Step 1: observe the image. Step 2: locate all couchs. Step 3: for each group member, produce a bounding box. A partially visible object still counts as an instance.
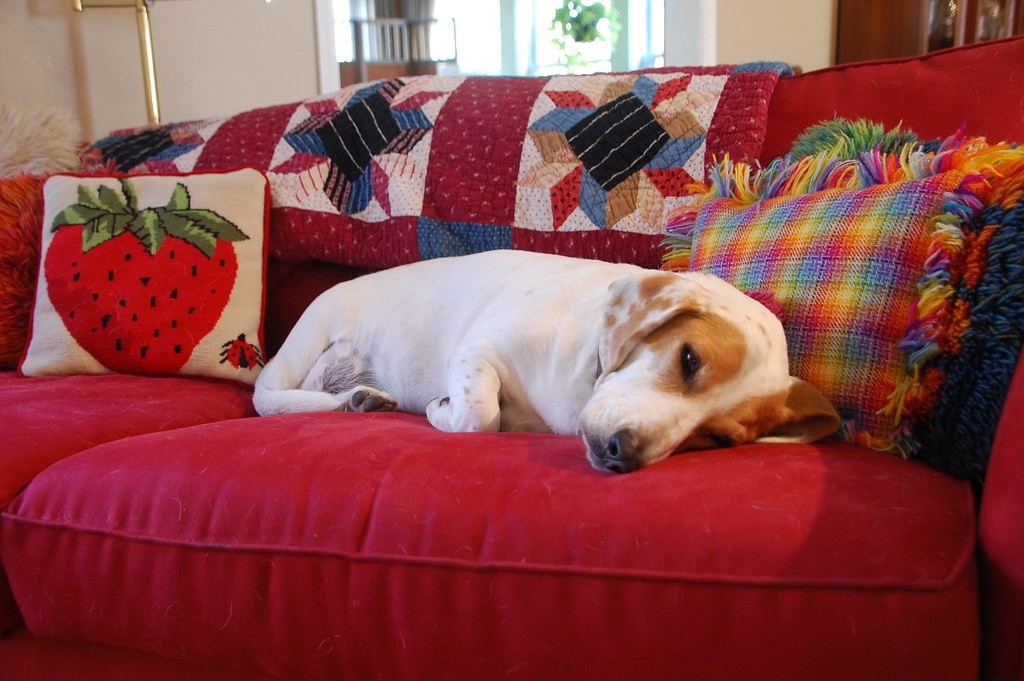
[0,36,1024,679]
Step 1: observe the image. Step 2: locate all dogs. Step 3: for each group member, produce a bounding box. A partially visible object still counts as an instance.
[251,249,841,472]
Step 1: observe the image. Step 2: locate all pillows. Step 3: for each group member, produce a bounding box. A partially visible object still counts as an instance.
[653,133,1024,457]
[18,170,272,394]
